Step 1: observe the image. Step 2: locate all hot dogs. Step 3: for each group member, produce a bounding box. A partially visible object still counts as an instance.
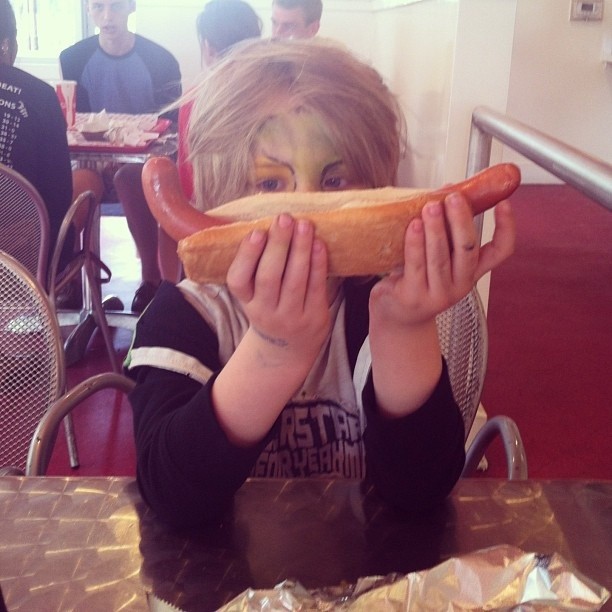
[142,158,519,285]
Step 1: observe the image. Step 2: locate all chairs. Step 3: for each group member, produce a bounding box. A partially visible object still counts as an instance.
[1,246,138,476]
[0,164,122,373]
[434,285,530,481]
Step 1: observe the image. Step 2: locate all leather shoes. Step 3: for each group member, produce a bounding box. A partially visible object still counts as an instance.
[131,278,162,313]
[56,280,84,310]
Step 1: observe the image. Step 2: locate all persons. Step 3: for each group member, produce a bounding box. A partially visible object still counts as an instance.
[270,0,323,40]
[57,0,183,312]
[196,0,264,69]
[0,1,75,301]
[124,38,516,509]
